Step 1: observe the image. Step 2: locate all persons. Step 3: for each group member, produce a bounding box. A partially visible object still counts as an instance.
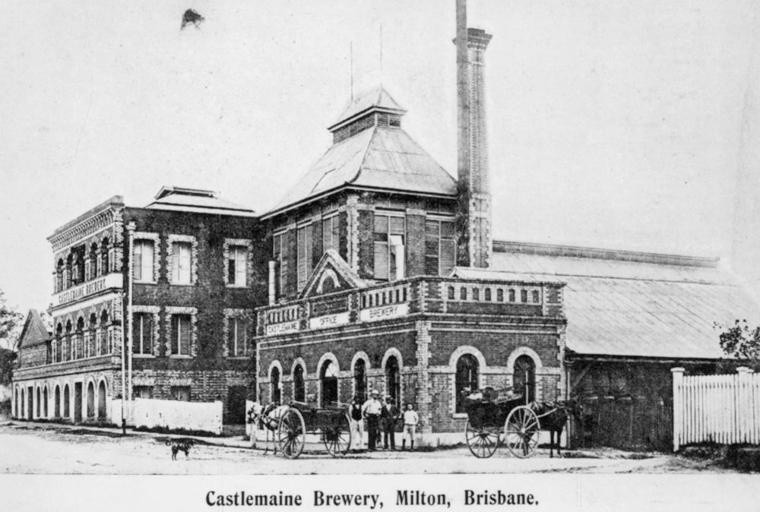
[362,390,383,452]
[402,403,418,451]
[349,396,364,451]
[381,395,400,451]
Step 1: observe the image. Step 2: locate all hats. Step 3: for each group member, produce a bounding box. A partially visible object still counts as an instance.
[386,395,393,400]
[372,390,379,394]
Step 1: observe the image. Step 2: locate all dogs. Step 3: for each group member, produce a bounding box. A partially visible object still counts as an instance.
[165,438,198,461]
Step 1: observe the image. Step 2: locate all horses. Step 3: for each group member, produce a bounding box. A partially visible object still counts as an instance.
[522,395,583,458]
[248,403,291,456]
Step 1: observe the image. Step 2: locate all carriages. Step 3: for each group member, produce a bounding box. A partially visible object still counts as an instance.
[245,397,353,458]
[460,382,582,459]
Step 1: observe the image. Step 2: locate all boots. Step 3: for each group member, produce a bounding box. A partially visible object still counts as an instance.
[411,440,414,450]
[402,439,405,450]
